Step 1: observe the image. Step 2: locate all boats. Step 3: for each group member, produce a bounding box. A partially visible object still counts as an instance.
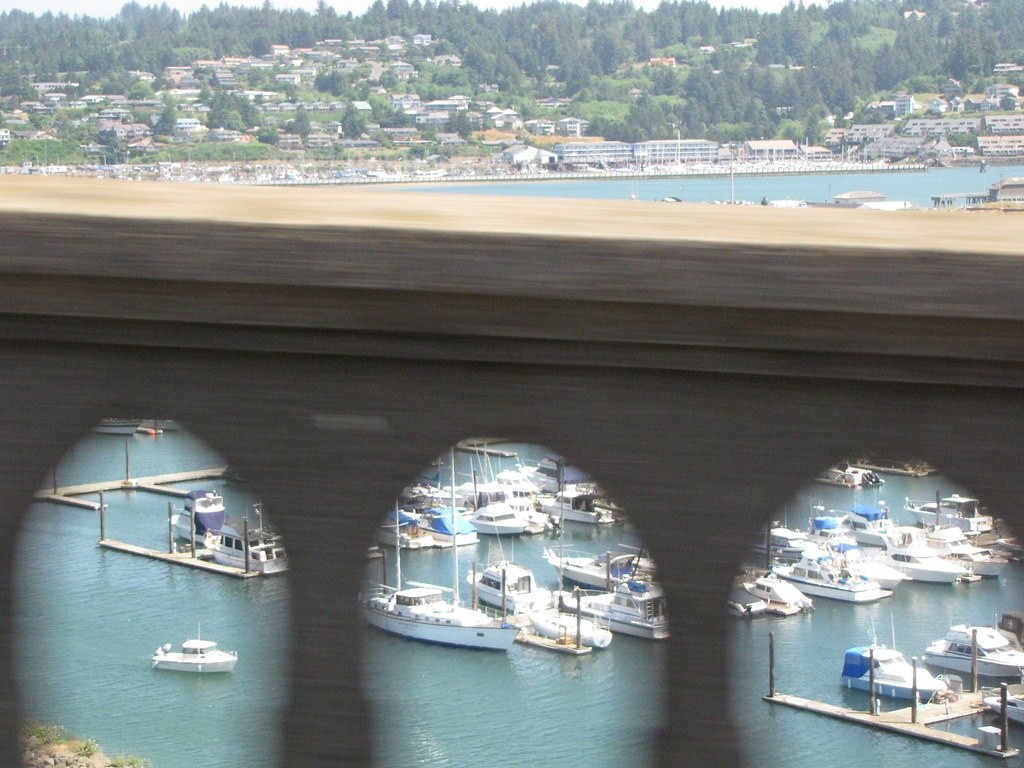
[730,453,1023,758]
[91,418,182,436]
[167,464,290,577]
[151,622,239,674]
[355,434,671,655]
